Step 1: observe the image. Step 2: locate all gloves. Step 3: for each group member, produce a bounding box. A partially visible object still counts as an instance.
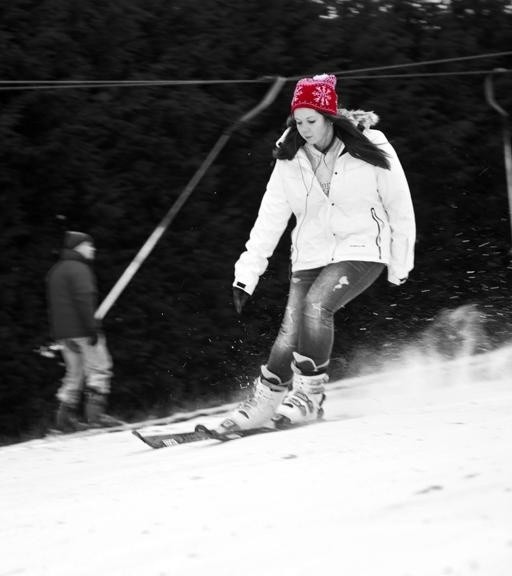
[231,288,249,314]
[85,330,97,346]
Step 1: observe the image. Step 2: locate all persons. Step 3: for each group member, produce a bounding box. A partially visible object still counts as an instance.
[39,227,126,438]
[219,68,420,436]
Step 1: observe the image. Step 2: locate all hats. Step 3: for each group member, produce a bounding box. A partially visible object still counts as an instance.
[291,73,337,116]
[62,230,91,250]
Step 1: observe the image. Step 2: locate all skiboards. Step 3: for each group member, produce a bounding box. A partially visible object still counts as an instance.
[132,417,328,449]
[42,404,228,447]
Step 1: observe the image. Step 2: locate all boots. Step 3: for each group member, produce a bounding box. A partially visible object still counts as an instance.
[214,364,292,438]
[80,392,125,425]
[271,351,331,429]
[45,400,86,433]
